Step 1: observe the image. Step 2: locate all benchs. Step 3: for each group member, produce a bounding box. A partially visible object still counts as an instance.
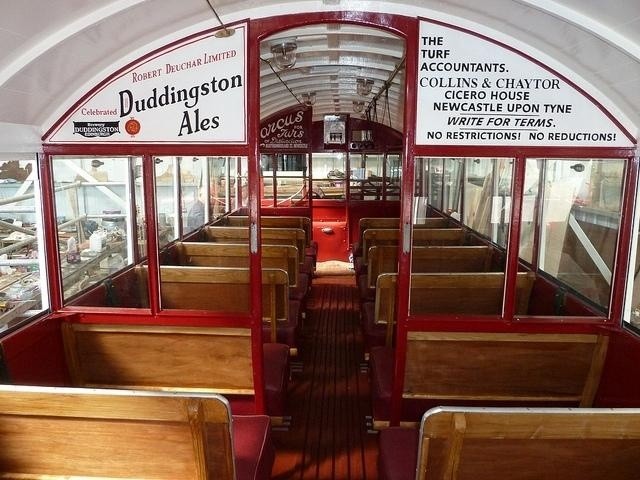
[368,316,619,424]
[374,403,640,478]
[0,379,277,476]
[349,217,494,300]
[129,260,303,350]
[200,215,319,281]
[61,301,295,420]
[358,270,536,343]
[173,238,309,306]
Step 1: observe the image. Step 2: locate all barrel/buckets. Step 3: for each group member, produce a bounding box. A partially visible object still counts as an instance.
[90,230,107,252]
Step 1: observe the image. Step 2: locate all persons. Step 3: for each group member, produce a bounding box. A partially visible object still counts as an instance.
[187,178,217,230]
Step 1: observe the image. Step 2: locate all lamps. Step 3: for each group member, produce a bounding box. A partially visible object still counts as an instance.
[355,77,374,97]
[269,40,297,69]
[300,94,315,108]
[354,102,364,114]
